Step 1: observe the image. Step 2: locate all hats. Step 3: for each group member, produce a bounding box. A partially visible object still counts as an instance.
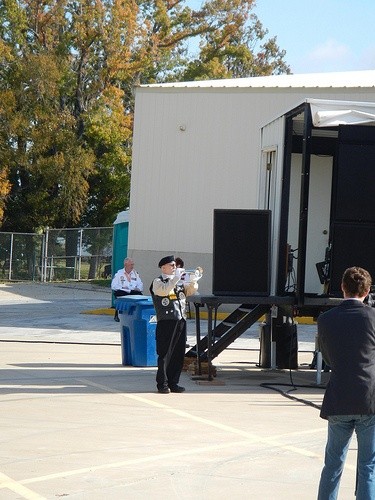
[158,256,174,269]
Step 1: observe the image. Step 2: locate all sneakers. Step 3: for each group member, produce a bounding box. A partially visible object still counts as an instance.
[158,386,171,394]
[173,386,186,393]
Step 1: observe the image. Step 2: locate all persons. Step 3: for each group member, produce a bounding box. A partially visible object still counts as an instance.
[174,257,190,348]
[317,266,375,500]
[111,257,143,322]
[149,255,203,393]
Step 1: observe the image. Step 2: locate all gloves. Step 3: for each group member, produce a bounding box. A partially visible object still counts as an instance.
[175,267,186,278]
[190,267,203,283]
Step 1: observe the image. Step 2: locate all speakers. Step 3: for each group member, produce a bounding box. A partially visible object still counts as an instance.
[259,323,298,369]
[327,124,375,298]
[212,209,272,296]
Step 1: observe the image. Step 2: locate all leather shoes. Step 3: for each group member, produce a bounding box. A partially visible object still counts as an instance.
[113,316,121,323]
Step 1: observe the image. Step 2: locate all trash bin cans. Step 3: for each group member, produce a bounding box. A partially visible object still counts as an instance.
[114,294,159,367]
